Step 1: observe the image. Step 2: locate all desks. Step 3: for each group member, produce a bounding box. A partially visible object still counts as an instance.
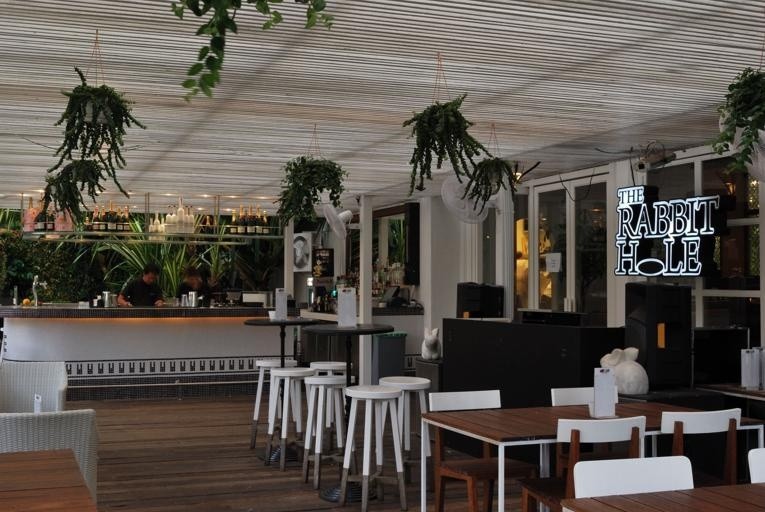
[242,318,321,463]
[561,483,764,512]
[302,323,394,503]
[2,449,97,511]
[422,402,763,501]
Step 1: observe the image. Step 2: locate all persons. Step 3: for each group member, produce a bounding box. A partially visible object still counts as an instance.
[117,263,165,308]
[175,265,206,306]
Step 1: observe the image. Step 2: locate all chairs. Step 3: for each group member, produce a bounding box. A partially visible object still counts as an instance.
[1,360,68,411]
[573,454,697,498]
[551,386,619,477]
[1,411,101,504]
[748,448,765,484]
[516,415,647,512]
[428,390,537,512]
[661,408,742,484]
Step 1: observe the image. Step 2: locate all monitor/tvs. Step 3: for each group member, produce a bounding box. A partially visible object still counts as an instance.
[379,286,400,303]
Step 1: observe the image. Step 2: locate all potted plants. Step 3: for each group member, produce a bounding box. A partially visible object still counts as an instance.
[402,51,522,216]
[37,31,151,232]
[273,124,351,226]
[229,206,270,234]
[703,37,765,182]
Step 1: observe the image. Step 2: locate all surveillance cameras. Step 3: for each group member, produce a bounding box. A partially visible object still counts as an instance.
[633,151,676,173]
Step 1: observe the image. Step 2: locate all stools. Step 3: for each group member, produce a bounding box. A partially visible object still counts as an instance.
[250,359,431,512]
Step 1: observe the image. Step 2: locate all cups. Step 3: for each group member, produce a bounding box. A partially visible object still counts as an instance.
[111,295,117,306]
[102,291,111,307]
[172,297,179,307]
[189,291,198,307]
[268,311,276,319]
[180,294,189,307]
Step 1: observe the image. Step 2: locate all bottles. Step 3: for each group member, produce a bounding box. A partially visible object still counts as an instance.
[149,205,194,233]
[84,205,130,231]
[312,294,333,313]
[229,203,270,235]
[33,209,55,230]
[334,272,383,296]
[205,215,212,241]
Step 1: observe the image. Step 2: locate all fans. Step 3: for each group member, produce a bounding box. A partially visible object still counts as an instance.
[440,176,502,224]
[321,203,353,240]
